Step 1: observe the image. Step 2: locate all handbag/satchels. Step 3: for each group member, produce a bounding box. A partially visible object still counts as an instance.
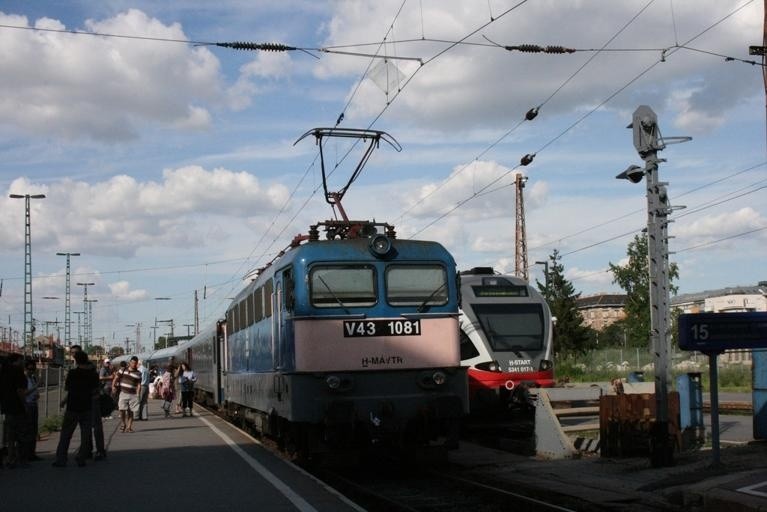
[110,386,120,401]
[100,394,116,416]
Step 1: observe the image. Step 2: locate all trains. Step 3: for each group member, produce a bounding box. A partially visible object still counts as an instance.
[108,219,468,467]
[455,265,555,412]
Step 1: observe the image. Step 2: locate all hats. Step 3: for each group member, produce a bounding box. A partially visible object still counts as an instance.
[104,359,110,363]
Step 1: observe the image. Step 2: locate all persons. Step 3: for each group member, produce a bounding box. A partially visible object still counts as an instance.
[608,378,625,395]
[1,346,198,467]
[228,334,275,373]
